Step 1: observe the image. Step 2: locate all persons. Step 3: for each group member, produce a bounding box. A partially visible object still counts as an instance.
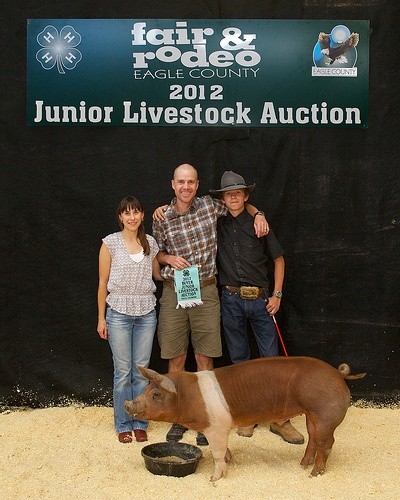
[97,196,164,443]
[153,171,305,444]
[151,164,269,445]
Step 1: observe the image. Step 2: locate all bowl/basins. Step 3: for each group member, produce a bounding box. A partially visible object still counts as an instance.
[141,442,202,477]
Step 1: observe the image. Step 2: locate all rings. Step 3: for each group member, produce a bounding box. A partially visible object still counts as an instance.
[264,228,267,230]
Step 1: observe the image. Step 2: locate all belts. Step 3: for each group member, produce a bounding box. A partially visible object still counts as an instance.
[223,285,269,300]
[164,278,215,287]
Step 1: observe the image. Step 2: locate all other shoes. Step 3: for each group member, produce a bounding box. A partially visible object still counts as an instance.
[270,419,304,443]
[118,429,133,443]
[238,424,256,438]
[134,429,147,442]
[167,422,188,442]
[197,430,208,446]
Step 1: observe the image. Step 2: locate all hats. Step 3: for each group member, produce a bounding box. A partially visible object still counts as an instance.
[209,171,257,192]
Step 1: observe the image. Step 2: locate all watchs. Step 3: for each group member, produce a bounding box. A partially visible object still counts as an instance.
[274,291,282,298]
[254,211,262,220]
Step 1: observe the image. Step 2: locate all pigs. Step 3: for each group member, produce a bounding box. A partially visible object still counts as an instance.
[122,356,366,482]
